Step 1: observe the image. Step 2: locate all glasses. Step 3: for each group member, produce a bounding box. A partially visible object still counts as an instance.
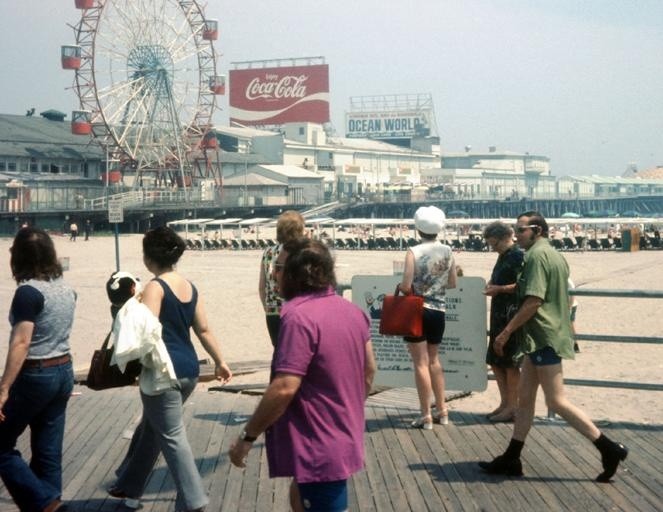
[518,225,537,232]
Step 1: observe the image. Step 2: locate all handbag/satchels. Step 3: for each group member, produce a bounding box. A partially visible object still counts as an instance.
[380,283,425,336]
[86,330,142,390]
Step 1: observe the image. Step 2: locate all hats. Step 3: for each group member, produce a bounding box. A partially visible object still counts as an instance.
[415,205,446,234]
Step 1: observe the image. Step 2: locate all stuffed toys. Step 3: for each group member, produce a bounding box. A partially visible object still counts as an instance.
[106,269,141,317]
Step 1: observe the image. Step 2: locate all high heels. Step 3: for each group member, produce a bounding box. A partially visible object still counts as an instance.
[412,409,448,429]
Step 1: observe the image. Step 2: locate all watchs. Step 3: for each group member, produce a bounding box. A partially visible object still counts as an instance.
[239,428,256,443]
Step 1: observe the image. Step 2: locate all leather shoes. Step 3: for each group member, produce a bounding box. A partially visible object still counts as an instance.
[597,442,627,481]
[479,456,522,474]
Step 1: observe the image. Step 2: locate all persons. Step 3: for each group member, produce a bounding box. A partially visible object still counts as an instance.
[481,212,631,483]
[550,218,661,245]
[214,231,218,243]
[204,229,208,241]
[229,243,374,512]
[481,222,528,425]
[67,220,78,240]
[0,226,77,511]
[256,209,310,350]
[405,202,458,432]
[108,228,232,511]
[83,220,91,240]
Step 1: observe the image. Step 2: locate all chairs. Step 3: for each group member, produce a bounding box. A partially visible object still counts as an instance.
[185,234,661,252]
